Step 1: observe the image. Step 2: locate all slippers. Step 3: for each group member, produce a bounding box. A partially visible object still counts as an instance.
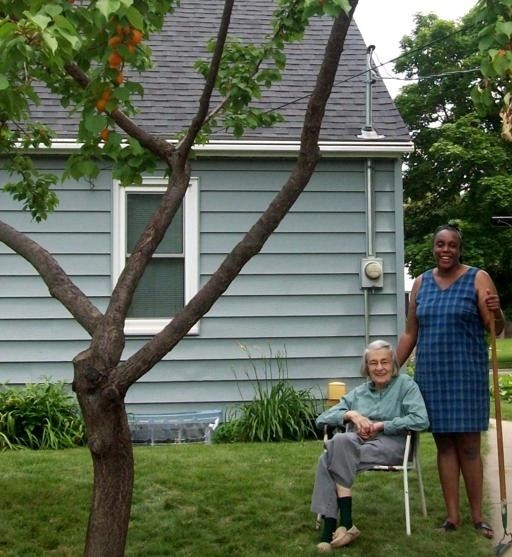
[472,521,493,540]
[439,520,462,534]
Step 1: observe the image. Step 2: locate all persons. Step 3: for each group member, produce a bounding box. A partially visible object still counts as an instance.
[395,224,505,539]
[310,340,430,551]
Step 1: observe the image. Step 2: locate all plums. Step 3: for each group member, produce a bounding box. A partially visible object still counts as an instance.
[95,23,142,141]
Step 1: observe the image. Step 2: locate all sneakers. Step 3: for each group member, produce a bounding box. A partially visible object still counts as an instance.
[317,524,362,553]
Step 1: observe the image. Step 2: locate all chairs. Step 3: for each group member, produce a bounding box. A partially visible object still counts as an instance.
[315,420,427,537]
